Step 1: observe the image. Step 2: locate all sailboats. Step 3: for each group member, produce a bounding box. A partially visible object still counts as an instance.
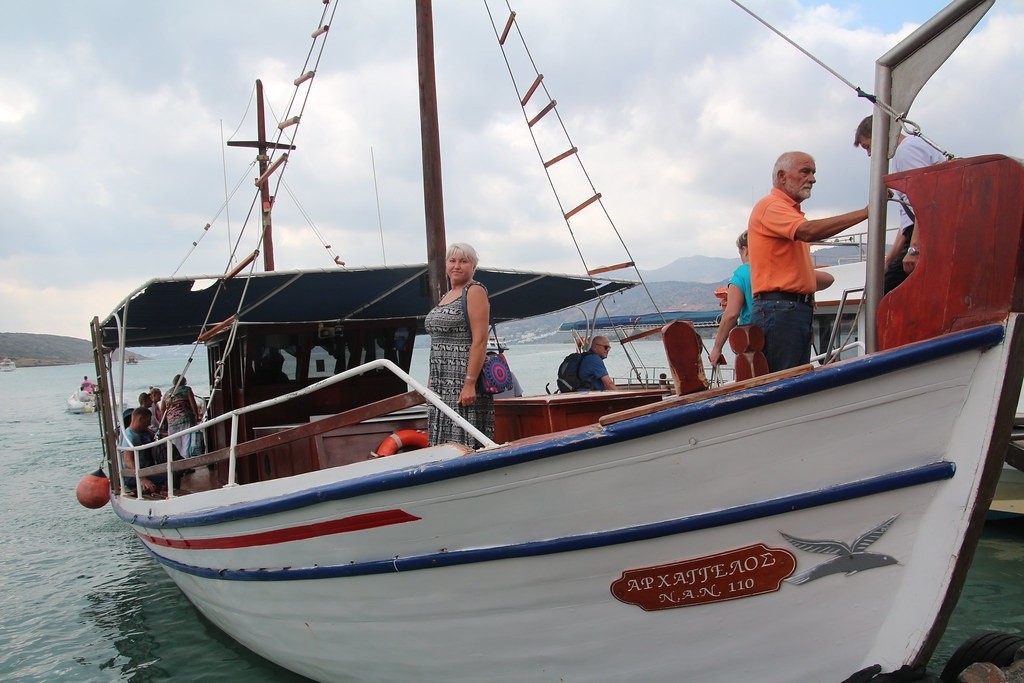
[73,0,1023,682]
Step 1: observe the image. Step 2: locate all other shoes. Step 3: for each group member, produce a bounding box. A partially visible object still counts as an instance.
[186,468,195,474]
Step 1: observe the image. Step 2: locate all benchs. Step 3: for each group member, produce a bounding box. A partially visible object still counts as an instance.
[136,484,189,500]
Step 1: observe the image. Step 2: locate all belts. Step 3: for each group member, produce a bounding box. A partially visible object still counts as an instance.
[754,290,814,307]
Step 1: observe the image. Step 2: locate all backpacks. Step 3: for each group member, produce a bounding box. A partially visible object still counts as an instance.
[557,352,596,393]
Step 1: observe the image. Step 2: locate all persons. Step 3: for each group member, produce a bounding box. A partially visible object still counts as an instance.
[557,336,616,393]
[81,376,92,393]
[855,115,946,294]
[709,230,834,367]
[747,152,894,373]
[79,387,90,402]
[120,375,199,494]
[486,352,524,398]
[425,243,494,452]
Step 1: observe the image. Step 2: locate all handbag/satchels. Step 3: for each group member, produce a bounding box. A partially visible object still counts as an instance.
[152,442,185,465]
[476,353,513,395]
[188,420,205,456]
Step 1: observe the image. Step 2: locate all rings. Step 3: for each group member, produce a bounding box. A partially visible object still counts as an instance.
[471,401,473,403]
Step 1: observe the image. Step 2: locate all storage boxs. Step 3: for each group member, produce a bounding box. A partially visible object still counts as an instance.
[252,414,433,481]
[493,390,670,444]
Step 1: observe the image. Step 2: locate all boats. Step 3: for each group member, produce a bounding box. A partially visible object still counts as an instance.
[66,391,98,415]
[0,357,17,373]
[557,220,1024,529]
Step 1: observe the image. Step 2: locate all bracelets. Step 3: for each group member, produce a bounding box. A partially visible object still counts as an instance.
[465,376,477,380]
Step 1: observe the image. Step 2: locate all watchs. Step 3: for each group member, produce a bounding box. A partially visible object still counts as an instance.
[908,248,920,255]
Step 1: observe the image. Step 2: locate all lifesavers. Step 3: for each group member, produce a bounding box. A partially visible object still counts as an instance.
[375,428,429,458]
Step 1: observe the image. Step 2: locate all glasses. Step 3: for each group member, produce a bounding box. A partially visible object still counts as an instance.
[596,343,611,350]
